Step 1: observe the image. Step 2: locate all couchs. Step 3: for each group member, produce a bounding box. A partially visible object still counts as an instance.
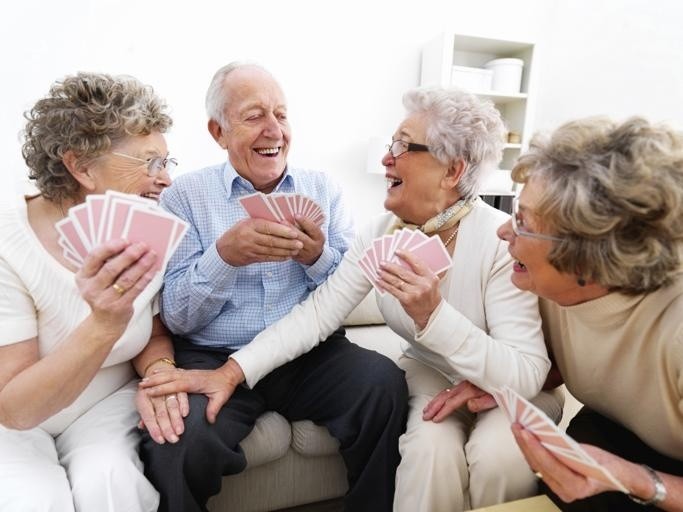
[209,287,401,512]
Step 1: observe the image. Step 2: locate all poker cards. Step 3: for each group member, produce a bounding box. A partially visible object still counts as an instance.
[358,227,453,296]
[54,189,190,276]
[236,193,327,227]
[489,386,629,493]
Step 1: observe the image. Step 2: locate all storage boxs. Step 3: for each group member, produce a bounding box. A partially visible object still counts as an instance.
[451,66,492,93]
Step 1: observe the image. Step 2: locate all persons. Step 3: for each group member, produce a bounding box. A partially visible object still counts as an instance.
[1,70,190,512]
[137,59,408,512]
[137,82,553,512]
[422,116,683,512]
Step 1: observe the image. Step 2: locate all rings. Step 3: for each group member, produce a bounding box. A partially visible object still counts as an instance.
[398,281,405,289]
[532,471,543,479]
[112,283,125,294]
[177,368,184,372]
[166,395,176,402]
[444,387,451,392]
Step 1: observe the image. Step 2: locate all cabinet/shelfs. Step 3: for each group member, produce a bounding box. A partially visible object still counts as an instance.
[421,31,536,212]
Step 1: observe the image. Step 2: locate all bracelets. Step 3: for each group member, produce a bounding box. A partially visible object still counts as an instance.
[144,357,176,373]
[627,464,666,506]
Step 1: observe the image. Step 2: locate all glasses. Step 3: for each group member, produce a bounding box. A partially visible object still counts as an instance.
[108,149,180,177]
[386,139,430,157]
[510,196,563,245]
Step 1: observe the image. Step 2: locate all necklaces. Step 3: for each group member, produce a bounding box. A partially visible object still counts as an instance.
[444,226,458,247]
